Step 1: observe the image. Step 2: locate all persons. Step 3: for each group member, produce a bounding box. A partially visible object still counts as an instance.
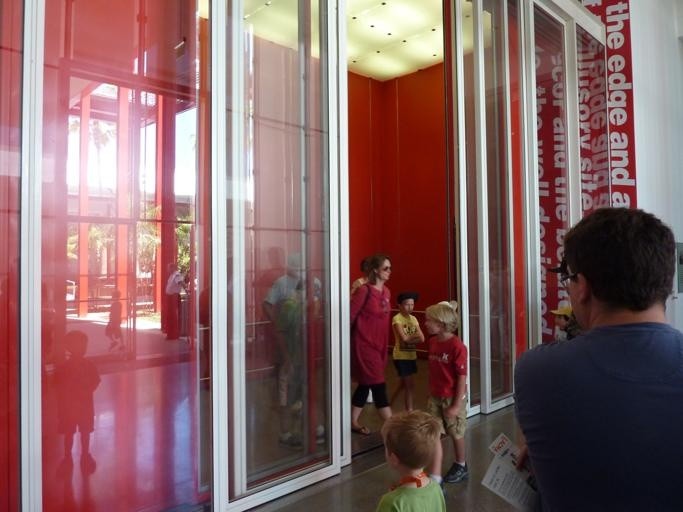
[515,207,682,512]
[548,256,580,345]
[52,330,101,472]
[160,263,191,342]
[350,254,468,512]
[101,291,131,351]
[261,242,322,445]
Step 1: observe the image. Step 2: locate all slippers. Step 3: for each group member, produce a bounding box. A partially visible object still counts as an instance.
[352,425,371,436]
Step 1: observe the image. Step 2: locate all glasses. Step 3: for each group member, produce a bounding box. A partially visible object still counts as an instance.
[381,266,392,272]
[559,273,577,294]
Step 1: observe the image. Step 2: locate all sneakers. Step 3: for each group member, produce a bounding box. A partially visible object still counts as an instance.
[366,390,372,404]
[443,461,469,483]
[108,341,125,351]
[55,453,96,473]
[439,482,447,499]
[278,428,326,449]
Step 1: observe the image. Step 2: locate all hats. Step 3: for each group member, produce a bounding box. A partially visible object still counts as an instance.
[397,292,419,304]
[549,305,572,319]
